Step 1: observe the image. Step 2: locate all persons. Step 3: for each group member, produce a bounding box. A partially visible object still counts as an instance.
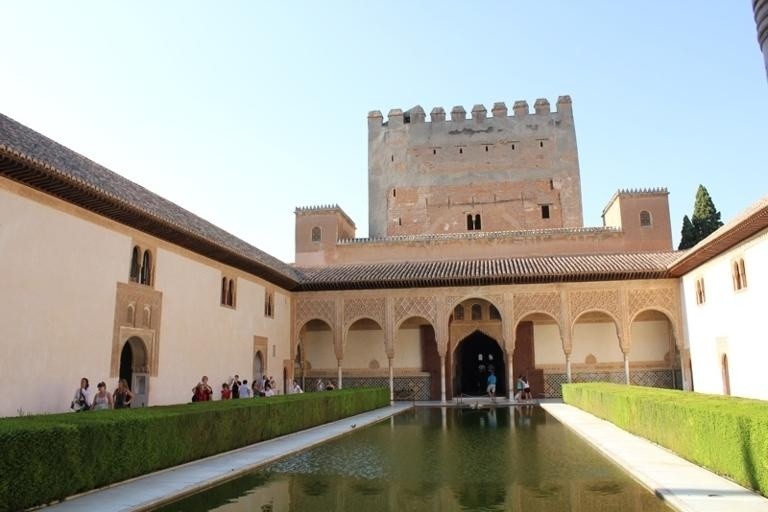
[317,379,324,392]
[291,380,303,394]
[522,376,533,400]
[70,377,93,411]
[191,373,276,403]
[113,379,135,408]
[326,380,334,391]
[90,382,114,410]
[514,375,526,400]
[486,372,497,399]
